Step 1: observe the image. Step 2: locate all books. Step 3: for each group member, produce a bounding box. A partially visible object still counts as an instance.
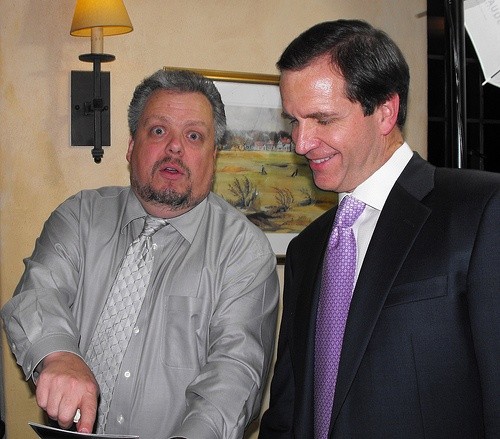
[28,421,140,439]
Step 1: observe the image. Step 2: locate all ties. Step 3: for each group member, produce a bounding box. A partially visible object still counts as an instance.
[314,195,365,439]
[79,217,169,436]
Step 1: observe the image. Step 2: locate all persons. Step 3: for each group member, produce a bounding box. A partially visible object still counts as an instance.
[259,20,500,439]
[0,70,280,439]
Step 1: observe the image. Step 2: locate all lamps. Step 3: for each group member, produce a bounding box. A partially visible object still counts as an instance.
[70,0,133,163]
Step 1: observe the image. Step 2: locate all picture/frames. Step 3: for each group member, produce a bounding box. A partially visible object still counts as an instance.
[164,66,339,265]
